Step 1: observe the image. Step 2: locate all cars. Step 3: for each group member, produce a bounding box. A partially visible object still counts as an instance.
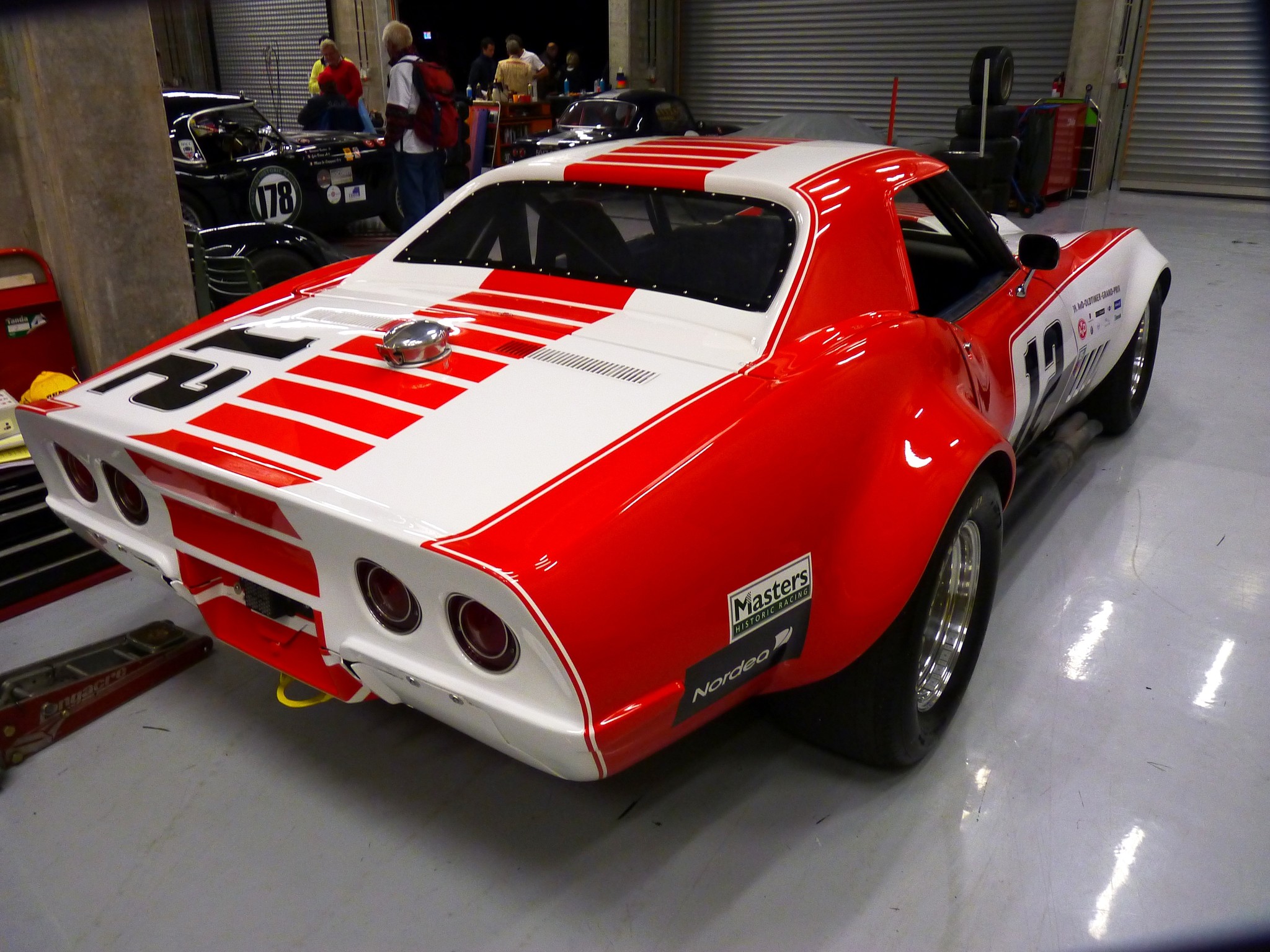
[513,89,742,162]
[186,219,354,319]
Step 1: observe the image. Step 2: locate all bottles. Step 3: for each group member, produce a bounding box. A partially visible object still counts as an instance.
[476,82,482,97]
[466,84,472,100]
[564,77,570,95]
[1052,72,1066,98]
[600,78,605,92]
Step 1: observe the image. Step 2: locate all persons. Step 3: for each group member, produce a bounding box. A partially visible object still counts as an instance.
[317,37,363,111]
[307,35,357,97]
[556,52,591,95]
[534,41,561,102]
[464,38,498,102]
[294,73,366,133]
[489,40,534,103]
[381,20,446,238]
[504,35,549,103]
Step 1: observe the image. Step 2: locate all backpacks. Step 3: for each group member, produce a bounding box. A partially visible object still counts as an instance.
[396,56,462,149]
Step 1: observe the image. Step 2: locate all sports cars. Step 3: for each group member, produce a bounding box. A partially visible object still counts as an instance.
[15,133,1175,790]
[163,89,443,242]
